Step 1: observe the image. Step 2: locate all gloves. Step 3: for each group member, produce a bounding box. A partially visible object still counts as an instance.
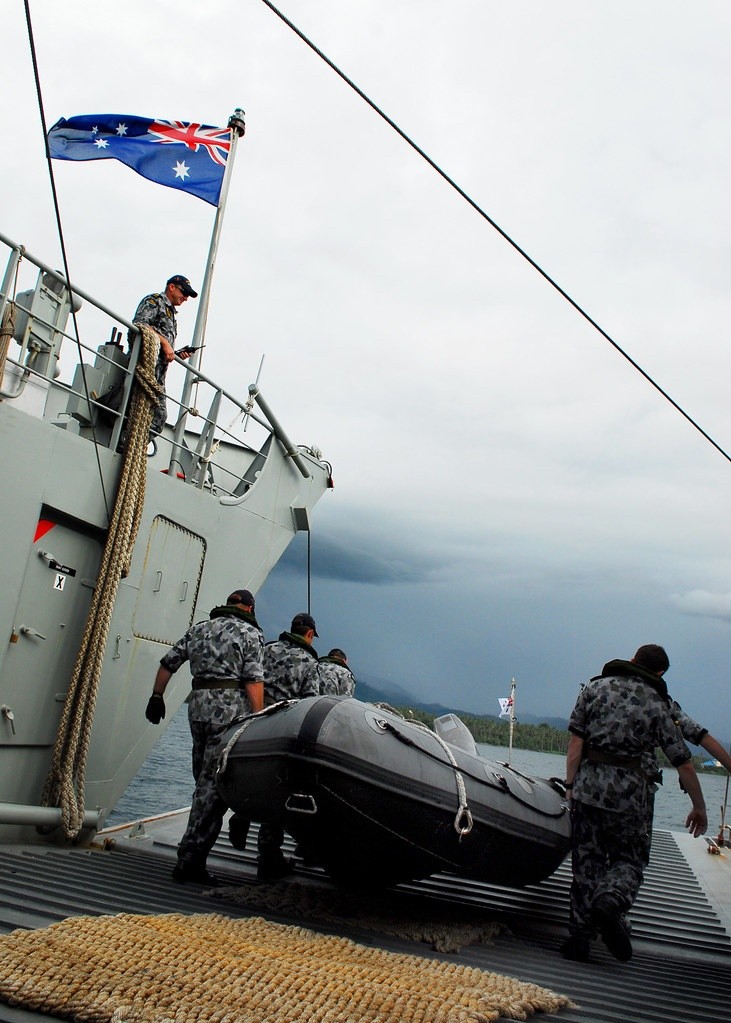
[146,691,165,725]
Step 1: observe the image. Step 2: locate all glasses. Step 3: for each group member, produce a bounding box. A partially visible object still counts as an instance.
[175,285,189,297]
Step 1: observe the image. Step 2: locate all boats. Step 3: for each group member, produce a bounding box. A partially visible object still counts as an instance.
[212,687,572,888]
[0,100,343,849]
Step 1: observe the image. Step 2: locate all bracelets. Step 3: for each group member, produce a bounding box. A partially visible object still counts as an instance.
[565,782,573,789]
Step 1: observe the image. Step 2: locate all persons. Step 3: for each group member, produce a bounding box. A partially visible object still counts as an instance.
[144,589,358,885]
[565,643,731,963]
[117,274,198,453]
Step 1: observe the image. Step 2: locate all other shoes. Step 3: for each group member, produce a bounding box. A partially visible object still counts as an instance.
[589,899,632,962]
[561,936,591,963]
[258,854,294,878]
[228,814,250,850]
[172,853,218,885]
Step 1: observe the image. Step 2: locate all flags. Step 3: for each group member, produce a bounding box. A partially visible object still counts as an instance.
[497,697,513,718]
[44,112,232,207]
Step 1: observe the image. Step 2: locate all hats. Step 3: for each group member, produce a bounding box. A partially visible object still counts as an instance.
[167,275,197,298]
[292,613,320,638]
[228,590,255,615]
[329,649,346,661]
